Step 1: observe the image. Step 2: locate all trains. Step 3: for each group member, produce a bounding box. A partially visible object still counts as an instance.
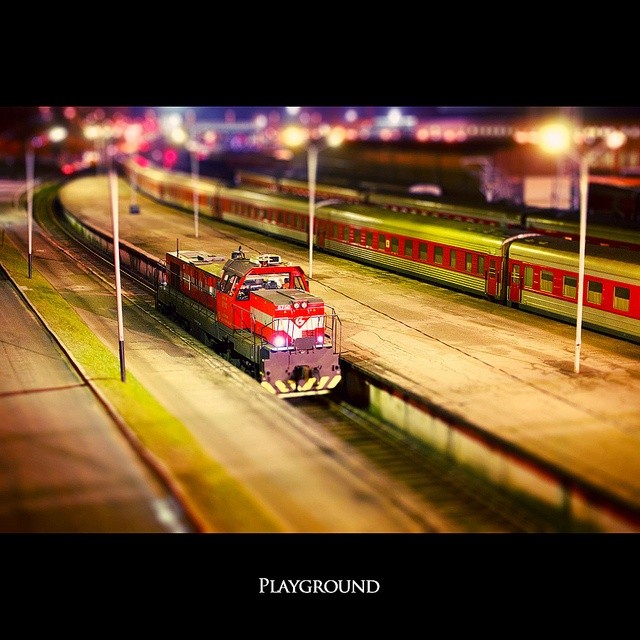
[157,240,343,401]
[114,151,639,345]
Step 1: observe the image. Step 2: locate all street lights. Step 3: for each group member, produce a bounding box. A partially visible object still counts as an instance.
[23,124,69,279]
[172,130,208,236]
[83,127,142,383]
[281,121,348,278]
[538,119,626,375]
